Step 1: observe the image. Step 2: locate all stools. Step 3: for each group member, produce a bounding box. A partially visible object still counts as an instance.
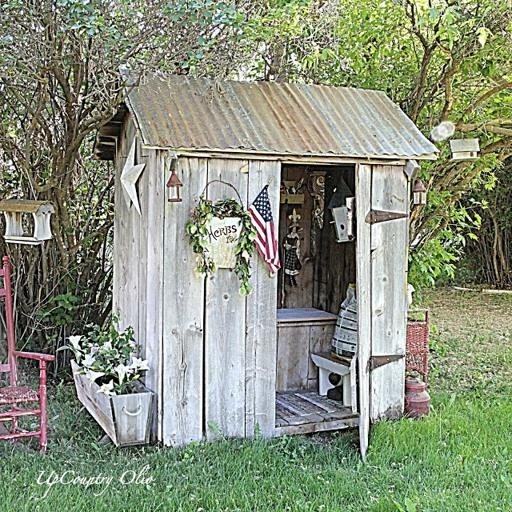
[308,351,351,410]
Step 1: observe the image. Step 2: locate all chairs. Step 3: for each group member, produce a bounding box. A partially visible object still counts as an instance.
[0,255,55,454]
[405,306,430,385]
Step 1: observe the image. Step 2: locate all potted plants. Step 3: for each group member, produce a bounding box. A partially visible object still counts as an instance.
[183,194,259,299]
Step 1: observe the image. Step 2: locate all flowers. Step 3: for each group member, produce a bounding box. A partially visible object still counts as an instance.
[54,307,150,397]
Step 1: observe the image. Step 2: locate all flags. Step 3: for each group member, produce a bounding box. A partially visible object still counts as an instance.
[244,186,282,276]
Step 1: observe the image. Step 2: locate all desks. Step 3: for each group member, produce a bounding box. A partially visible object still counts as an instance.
[277,306,338,393]
